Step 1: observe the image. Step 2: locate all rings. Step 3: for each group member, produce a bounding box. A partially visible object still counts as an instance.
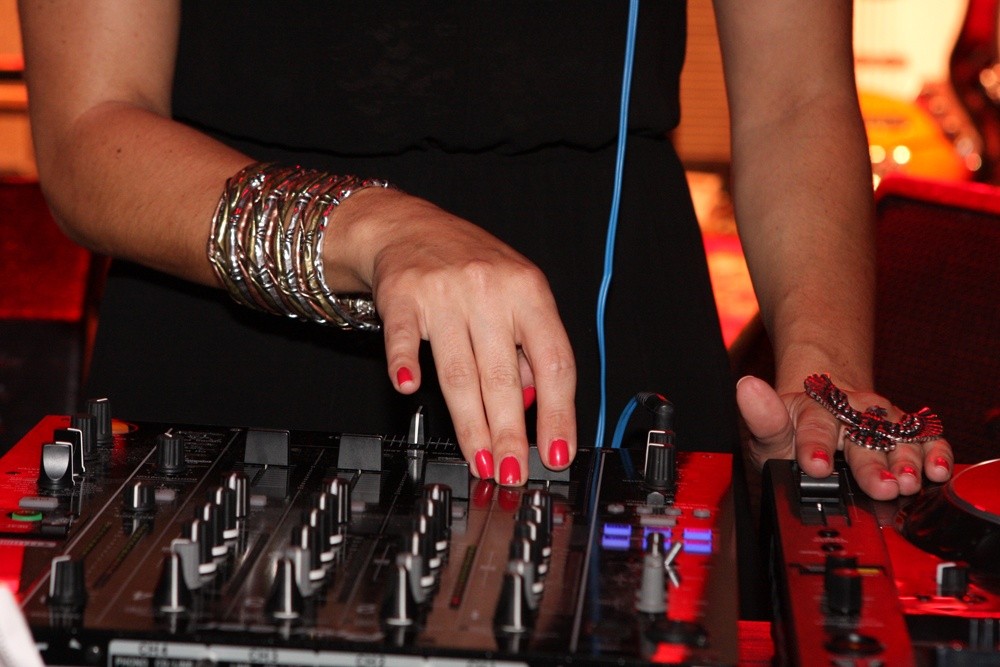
[803,372,943,452]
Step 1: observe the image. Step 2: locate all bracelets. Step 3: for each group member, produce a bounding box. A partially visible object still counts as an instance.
[206,161,404,332]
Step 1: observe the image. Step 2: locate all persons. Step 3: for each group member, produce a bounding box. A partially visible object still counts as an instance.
[15,0,954,500]
